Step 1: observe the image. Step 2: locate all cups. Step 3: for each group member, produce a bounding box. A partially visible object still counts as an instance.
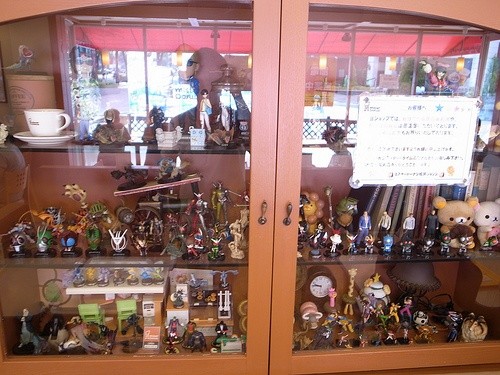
[24,108,71,137]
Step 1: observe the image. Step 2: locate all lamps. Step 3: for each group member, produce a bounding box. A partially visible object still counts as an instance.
[387,261,442,310]
[101,49,110,66]
[177,50,182,66]
[247,52,253,69]
[320,54,327,69]
[456,39,464,72]
[391,56,397,70]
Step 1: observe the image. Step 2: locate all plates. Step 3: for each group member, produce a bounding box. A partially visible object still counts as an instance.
[13,130,78,144]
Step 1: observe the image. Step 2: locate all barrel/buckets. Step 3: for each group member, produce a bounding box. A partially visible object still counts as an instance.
[5,74,57,132]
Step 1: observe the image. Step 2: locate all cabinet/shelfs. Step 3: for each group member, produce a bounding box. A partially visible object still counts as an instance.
[0,0,500,375]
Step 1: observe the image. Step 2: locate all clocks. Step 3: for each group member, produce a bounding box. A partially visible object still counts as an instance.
[300,265,336,310]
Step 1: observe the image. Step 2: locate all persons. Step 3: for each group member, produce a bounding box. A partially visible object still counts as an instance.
[199,89,213,132]
[321,127,352,168]
[188,192,208,235]
[308,210,437,251]
[312,297,412,347]
[169,317,181,333]
[211,182,235,224]
[165,47,249,222]
[215,321,228,339]
[228,208,249,253]
[423,64,447,89]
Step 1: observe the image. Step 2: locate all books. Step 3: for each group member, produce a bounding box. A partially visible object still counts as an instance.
[346,156,500,244]
